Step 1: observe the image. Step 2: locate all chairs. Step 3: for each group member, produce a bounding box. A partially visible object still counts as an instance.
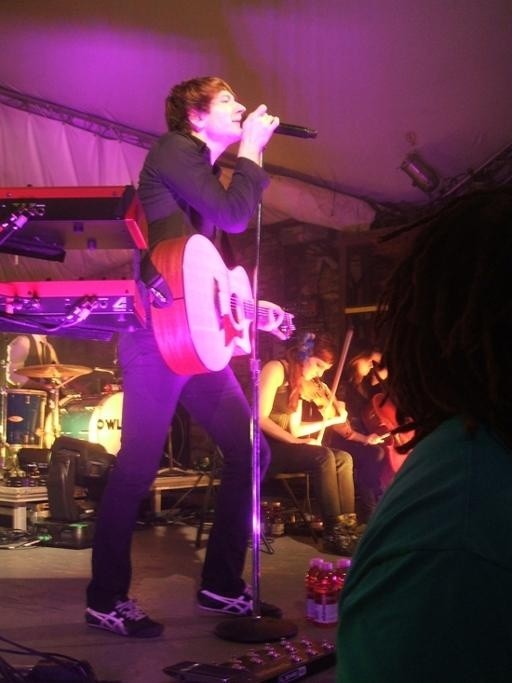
[195,434,321,554]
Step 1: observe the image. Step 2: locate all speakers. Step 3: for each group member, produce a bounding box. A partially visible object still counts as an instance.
[159,402,190,468]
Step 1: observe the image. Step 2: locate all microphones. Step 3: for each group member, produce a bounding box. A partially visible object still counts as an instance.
[238,115,319,139]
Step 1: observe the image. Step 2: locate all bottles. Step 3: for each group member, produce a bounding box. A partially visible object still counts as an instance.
[302,555,351,626]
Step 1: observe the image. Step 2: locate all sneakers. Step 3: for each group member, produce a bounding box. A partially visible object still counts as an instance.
[198,588,281,618]
[85,597,162,637]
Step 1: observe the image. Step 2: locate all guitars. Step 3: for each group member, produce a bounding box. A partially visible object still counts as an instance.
[146,235,293,377]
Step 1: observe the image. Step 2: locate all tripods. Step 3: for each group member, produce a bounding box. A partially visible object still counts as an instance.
[157,421,193,476]
[195,446,274,554]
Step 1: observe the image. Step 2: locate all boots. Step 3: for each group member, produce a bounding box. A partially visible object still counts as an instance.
[324,513,363,554]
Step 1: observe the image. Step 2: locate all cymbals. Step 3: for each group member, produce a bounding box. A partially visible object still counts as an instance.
[13,363,92,380]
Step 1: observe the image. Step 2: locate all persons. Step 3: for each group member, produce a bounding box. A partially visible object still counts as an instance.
[337,189,512,683]
[85,77,284,637]
[319,336,385,524]
[6,331,60,449]
[259,335,363,556]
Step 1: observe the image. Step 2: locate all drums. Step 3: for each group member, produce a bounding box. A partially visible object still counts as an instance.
[49,392,126,458]
[1,389,47,451]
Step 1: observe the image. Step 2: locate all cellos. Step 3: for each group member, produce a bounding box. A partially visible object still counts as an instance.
[357,342,424,492]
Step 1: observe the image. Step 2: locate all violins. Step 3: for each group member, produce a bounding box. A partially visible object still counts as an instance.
[302,379,347,422]
